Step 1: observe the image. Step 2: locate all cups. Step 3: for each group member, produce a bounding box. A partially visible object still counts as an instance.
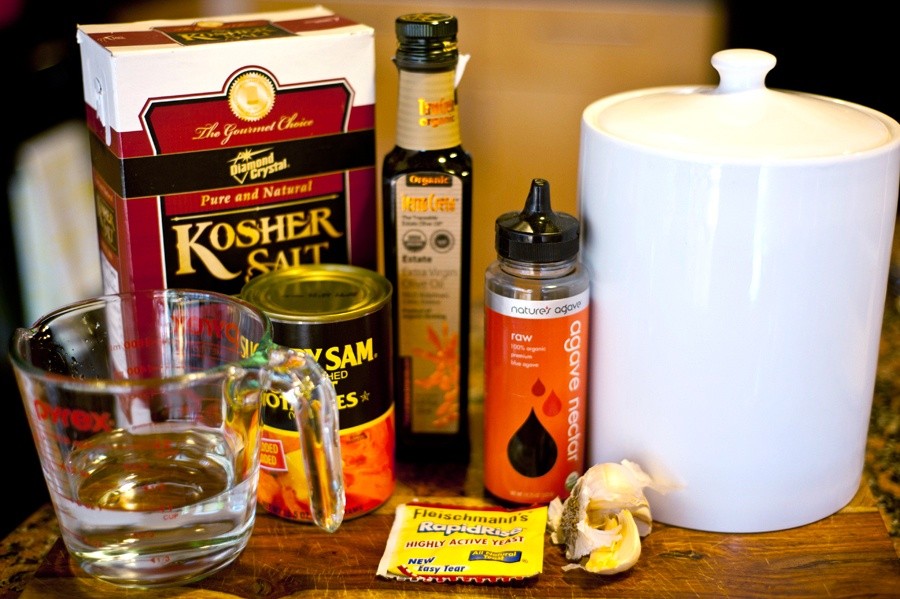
[8,288,345,592]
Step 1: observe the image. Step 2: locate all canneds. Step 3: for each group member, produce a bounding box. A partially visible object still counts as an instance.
[243,264,396,527]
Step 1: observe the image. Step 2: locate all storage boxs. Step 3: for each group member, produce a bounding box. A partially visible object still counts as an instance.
[77,5,379,426]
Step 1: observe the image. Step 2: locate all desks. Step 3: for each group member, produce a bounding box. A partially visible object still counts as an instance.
[1,408,900,599]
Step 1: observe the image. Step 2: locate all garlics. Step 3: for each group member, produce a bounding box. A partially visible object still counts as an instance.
[553,462,654,575]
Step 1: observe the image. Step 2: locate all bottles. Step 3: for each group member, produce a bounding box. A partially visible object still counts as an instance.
[577,48,900,535]
[484,177,591,503]
[380,10,473,461]
